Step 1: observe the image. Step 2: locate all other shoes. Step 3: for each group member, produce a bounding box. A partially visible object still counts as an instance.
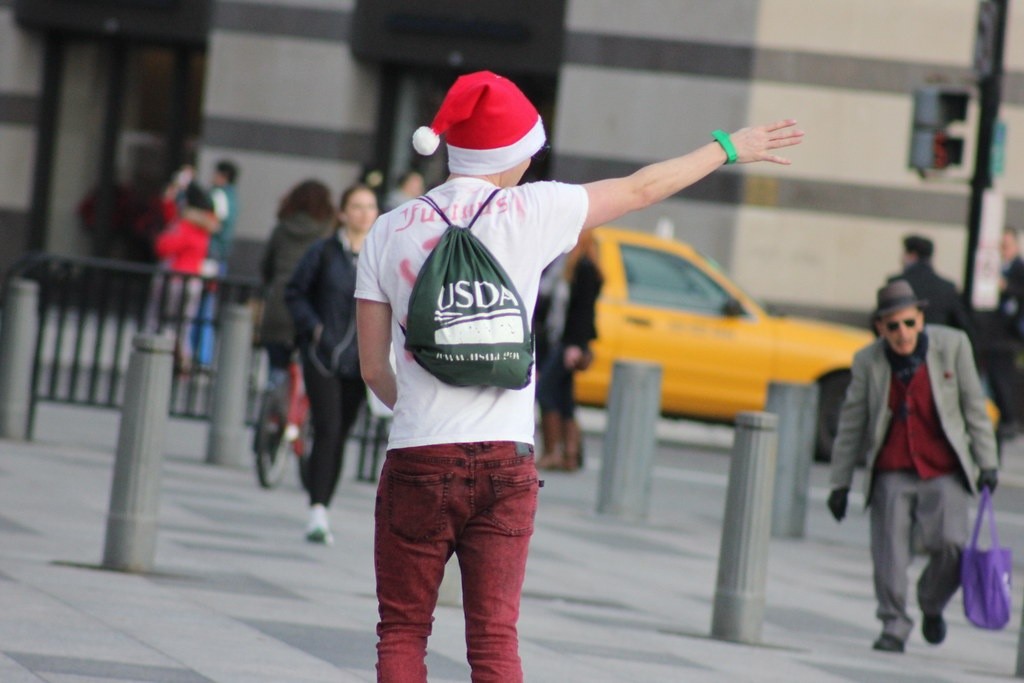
[923,607,947,643]
[305,502,335,545]
[873,634,902,652]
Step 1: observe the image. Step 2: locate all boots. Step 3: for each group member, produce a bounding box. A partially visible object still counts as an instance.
[563,418,580,471]
[534,412,564,472]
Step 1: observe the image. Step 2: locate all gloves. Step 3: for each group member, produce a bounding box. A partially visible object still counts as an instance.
[977,465,999,497]
[828,488,847,525]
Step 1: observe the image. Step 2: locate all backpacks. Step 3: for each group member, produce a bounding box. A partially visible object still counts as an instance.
[401,192,533,390]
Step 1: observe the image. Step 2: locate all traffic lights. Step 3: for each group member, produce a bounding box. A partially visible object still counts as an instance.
[911,82,970,174]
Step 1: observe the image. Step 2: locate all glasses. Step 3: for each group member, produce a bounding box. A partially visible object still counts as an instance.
[883,315,921,330]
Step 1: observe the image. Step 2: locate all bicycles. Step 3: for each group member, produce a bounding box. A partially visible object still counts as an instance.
[254,349,312,490]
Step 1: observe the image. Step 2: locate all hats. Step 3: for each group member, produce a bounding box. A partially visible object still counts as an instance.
[412,68,548,177]
[874,280,929,321]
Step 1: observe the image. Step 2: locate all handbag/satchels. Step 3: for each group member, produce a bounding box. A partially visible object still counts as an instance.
[958,490,1011,628]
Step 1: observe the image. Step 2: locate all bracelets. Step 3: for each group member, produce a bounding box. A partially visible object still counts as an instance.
[712,129,737,165]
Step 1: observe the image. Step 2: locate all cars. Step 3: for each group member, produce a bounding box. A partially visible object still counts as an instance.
[570,225,1002,469]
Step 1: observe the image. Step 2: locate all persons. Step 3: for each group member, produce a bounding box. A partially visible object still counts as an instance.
[998,226,1024,435]
[161,158,239,370]
[828,281,998,651]
[385,171,423,210]
[251,179,379,542]
[534,230,604,470]
[890,233,972,335]
[352,70,804,683]
